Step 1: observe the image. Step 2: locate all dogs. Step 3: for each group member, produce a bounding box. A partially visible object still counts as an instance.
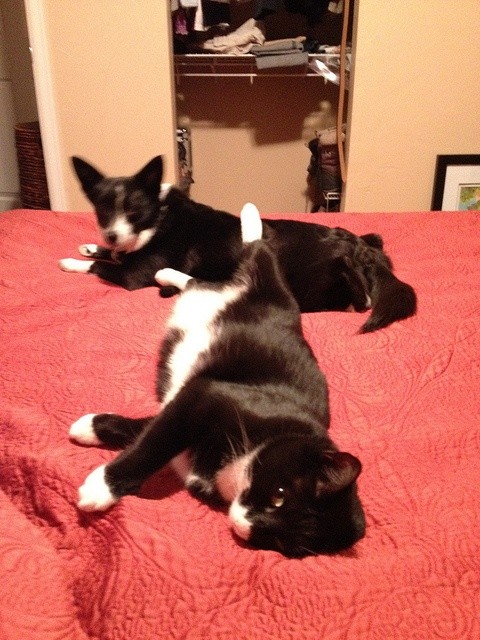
[57,155,419,338]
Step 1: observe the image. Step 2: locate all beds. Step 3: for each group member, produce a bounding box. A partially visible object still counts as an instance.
[0,207,479,640]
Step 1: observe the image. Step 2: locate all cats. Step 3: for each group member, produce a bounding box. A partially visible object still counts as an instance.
[65,202,367,559]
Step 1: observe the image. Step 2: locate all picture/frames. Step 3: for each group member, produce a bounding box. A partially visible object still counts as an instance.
[430,155,480,212]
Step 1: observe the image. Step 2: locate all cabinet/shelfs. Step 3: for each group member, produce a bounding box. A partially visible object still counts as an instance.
[175,48,353,82]
